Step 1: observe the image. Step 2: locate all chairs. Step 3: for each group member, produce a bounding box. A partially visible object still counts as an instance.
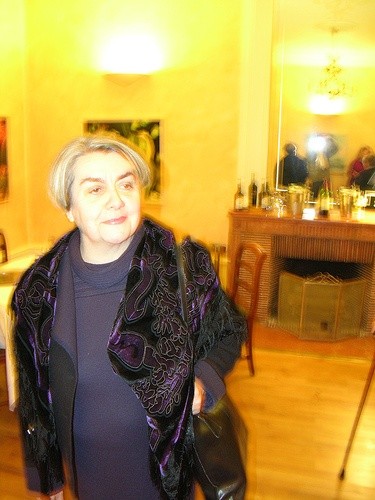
[182,235,266,375]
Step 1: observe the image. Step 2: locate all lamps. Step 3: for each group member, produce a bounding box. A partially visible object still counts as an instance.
[301,19,349,116]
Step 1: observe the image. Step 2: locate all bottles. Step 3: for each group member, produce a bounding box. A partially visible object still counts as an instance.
[320,179,329,216]
[234,179,244,212]
[248,173,258,208]
[259,183,270,209]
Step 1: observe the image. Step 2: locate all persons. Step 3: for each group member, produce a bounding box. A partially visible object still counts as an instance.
[314,150,333,198]
[10,138,251,500]
[355,154,375,190]
[275,143,308,188]
[346,146,370,188]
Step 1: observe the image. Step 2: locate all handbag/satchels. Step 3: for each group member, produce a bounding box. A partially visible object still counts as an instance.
[187,391,248,500]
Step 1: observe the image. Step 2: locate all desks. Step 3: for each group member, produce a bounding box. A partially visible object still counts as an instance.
[0,249,50,411]
[228,204,375,339]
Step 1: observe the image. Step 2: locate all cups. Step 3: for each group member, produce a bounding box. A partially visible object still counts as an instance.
[337,189,353,220]
[288,191,303,220]
